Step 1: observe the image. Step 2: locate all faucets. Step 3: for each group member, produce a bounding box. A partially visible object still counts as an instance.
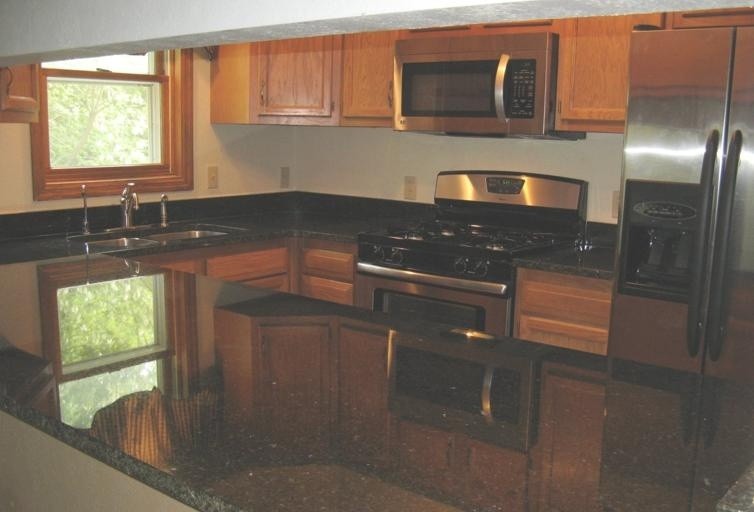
[125,193,140,215]
[79,184,89,221]
[160,194,169,225]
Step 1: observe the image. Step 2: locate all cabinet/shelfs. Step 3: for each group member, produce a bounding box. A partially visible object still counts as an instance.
[297,229,361,306]
[331,314,396,479]
[134,244,204,275]
[550,9,667,137]
[341,26,401,130]
[389,412,527,512]
[0,341,62,427]
[203,234,295,298]
[206,33,340,131]
[215,309,335,464]
[536,360,610,512]
[1,59,44,125]
[511,260,613,356]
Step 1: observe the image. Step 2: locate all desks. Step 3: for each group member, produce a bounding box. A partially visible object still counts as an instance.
[0,251,754,512]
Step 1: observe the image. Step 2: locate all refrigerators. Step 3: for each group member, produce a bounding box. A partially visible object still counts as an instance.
[596,358,753,512]
[611,28,753,381]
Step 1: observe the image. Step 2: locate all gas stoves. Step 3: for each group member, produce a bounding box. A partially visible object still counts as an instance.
[354,216,587,279]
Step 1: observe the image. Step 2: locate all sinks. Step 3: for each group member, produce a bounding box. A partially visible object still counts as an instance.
[147,229,227,239]
[86,237,159,253]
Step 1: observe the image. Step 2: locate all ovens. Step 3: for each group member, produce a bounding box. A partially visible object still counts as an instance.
[351,260,513,336]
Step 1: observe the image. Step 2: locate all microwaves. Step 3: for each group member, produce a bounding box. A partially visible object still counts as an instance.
[380,334,541,454]
[395,33,583,142]
